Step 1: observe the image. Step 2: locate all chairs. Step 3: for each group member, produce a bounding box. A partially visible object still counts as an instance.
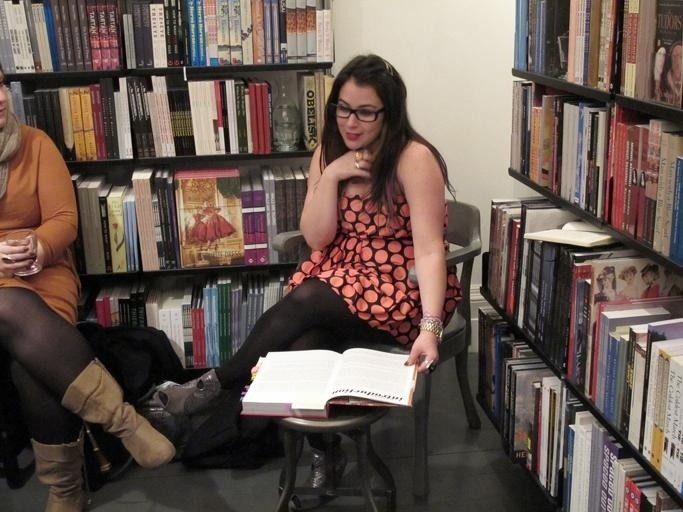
[0,175,96,489]
[272,202,482,501]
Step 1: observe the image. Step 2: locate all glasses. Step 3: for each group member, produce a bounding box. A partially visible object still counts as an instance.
[328,102,384,123]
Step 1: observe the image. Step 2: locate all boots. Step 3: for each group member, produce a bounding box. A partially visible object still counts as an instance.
[60,357,176,469]
[30,427,85,512]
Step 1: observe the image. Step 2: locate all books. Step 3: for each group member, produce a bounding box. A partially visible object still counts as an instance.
[491,195,680,489]
[240,348,421,417]
[1,0,334,70]
[478,307,683,512]
[74,271,294,366]
[10,73,335,159]
[496,76,683,261]
[69,159,310,267]
[513,0,682,107]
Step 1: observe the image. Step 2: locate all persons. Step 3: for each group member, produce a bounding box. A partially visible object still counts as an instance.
[1,67,182,512]
[154,54,464,512]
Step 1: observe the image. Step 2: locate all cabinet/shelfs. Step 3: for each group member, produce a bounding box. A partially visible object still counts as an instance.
[1,1,337,378]
[475,1,678,509]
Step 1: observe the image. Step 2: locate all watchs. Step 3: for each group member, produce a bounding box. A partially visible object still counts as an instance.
[420,319,444,344]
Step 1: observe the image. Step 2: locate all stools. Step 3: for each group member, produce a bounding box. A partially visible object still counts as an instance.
[275,405,400,509]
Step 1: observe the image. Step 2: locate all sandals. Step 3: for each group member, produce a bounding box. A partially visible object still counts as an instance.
[292,447,348,508]
[155,368,221,418]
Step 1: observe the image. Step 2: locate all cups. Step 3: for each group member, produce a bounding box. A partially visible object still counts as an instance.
[6,228,42,277]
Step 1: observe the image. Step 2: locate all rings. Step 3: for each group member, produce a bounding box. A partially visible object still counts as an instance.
[355,151,364,161]
[1,256,15,265]
[354,161,360,169]
[423,360,436,372]
